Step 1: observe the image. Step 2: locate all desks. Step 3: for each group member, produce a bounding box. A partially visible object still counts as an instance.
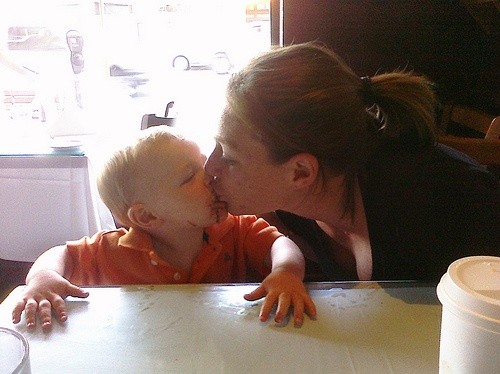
[0,279,443,374]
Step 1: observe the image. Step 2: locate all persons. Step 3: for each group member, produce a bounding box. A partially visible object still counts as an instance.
[203,44,500,281]
[11,125,317,328]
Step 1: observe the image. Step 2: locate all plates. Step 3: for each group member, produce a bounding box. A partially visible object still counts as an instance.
[49,142,83,151]
[0,326,30,373]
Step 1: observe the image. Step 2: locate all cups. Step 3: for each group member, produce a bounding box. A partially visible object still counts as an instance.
[436,256,500,374]
